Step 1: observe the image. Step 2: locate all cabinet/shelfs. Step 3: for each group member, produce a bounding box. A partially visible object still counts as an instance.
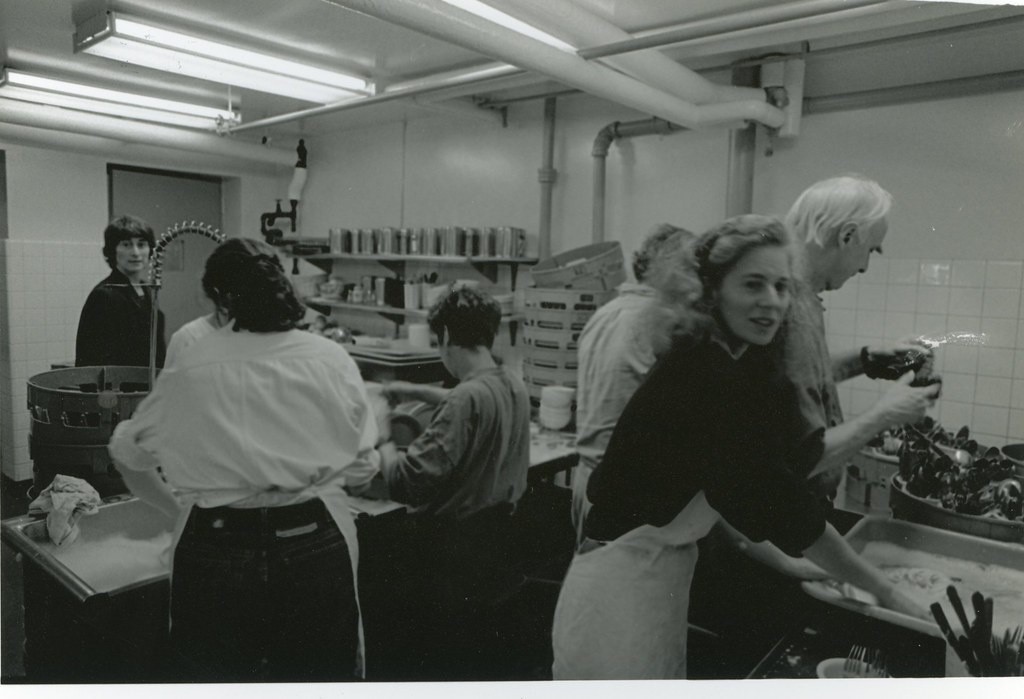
[302,254,540,350]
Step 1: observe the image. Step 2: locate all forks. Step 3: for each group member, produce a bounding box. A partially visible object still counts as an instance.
[842,645,866,677]
[931,585,1024,677]
[865,649,889,678]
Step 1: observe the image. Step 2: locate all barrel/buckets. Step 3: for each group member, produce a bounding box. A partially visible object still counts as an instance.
[841,450,901,514]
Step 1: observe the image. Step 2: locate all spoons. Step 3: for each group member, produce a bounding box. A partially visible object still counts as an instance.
[850,416,1024,522]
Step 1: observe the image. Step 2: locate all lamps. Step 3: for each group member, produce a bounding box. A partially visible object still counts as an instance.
[72,10,377,105]
[0,67,241,131]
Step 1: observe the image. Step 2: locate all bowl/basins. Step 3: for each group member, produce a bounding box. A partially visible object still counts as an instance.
[538,386,576,430]
[890,473,1024,543]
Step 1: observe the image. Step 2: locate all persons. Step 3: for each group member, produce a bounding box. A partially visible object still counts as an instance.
[696,175,940,679]
[366,282,532,682]
[107,238,382,683]
[74,214,167,367]
[568,222,697,547]
[549,216,935,679]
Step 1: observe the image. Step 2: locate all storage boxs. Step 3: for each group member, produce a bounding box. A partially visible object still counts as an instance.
[532,239,628,290]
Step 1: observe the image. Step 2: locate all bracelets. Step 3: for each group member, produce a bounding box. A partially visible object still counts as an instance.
[861,346,878,380]
[374,436,394,449]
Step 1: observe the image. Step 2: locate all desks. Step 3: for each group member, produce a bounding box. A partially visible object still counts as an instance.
[2,420,579,684]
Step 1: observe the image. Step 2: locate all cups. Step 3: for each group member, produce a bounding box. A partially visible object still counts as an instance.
[328,227,528,256]
[408,325,430,346]
[362,276,435,309]
